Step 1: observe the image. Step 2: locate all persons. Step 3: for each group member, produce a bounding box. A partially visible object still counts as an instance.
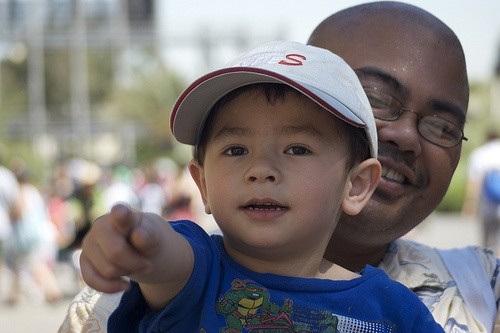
[0,134,225,310]
[80,41,434,333]
[55,0,500,333]
[458,124,500,256]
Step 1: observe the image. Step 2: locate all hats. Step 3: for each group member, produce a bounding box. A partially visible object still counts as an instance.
[169,39,378,160]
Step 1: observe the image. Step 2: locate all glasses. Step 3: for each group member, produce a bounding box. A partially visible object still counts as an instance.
[362,85,468,148]
